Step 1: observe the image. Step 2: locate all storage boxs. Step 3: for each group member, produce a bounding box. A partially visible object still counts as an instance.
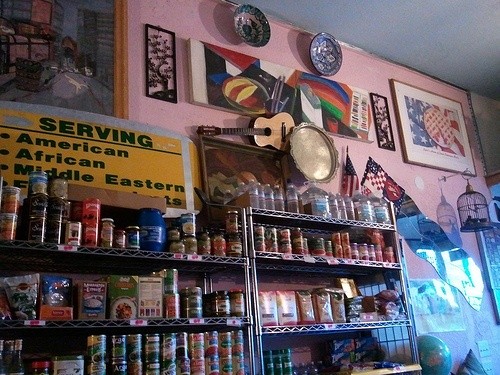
[38,274,163,319]
[259,290,296,326]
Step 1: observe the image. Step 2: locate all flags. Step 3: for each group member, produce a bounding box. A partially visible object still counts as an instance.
[343,153,359,196]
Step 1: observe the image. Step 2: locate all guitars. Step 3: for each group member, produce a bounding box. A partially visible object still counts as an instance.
[196,112,295,152]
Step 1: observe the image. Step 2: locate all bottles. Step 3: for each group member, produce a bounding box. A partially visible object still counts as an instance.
[138,207,168,252]
[221,178,392,224]
[262,348,324,375]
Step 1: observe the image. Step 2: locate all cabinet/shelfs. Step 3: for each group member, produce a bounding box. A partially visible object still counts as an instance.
[0,203,255,375]
[209,207,422,375]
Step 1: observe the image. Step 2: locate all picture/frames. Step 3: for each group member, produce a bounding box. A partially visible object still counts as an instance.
[198,135,290,210]
[390,78,478,177]
[0,0,130,120]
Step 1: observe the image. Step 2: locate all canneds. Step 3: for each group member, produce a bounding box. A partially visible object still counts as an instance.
[32,361,54,375]
[262,348,293,375]
[100,217,141,249]
[152,269,246,318]
[24,170,101,247]
[167,210,243,259]
[86,330,245,375]
[254,226,396,263]
[0,185,21,242]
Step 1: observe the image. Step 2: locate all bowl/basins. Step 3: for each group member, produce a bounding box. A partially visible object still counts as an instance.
[309,31,343,76]
[232,4,271,47]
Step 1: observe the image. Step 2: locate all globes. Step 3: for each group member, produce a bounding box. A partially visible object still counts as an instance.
[416,334,452,375]
